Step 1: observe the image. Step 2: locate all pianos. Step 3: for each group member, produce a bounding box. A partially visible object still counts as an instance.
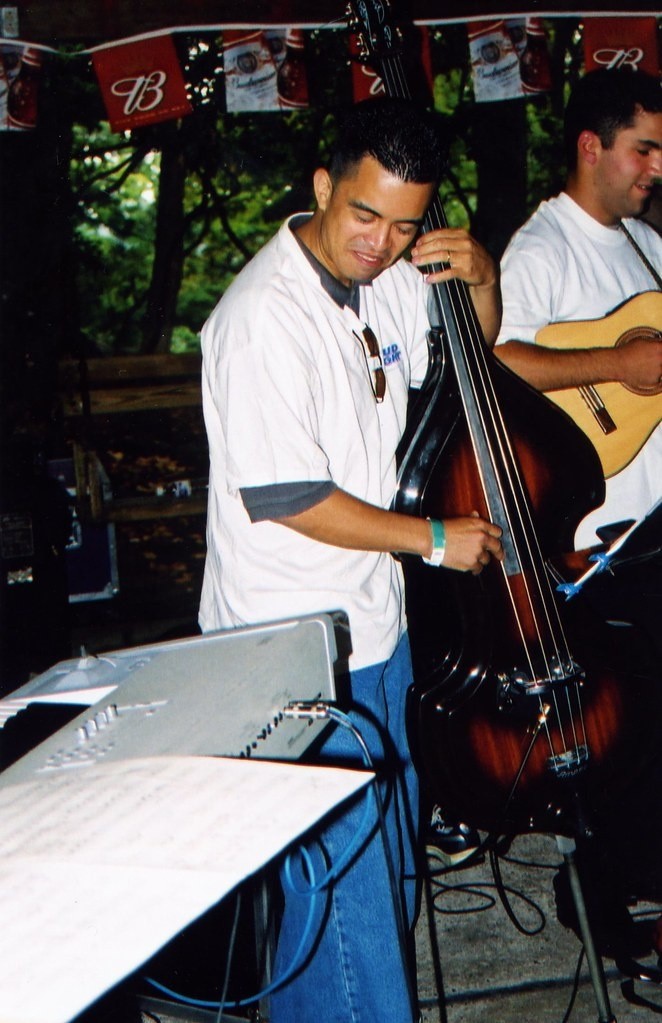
[1,611,348,1023]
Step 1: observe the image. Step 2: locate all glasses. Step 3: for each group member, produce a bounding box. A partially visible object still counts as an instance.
[352,323,386,404]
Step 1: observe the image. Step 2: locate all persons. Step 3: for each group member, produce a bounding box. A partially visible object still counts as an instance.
[491,66,662,957]
[196,96,504,1023]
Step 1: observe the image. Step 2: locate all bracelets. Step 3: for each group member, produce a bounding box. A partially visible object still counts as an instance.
[422,516,445,567]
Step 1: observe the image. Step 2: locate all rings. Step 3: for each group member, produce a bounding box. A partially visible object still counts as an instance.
[446,250,450,262]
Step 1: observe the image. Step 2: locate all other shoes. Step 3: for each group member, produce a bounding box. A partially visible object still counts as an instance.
[424,815,481,866]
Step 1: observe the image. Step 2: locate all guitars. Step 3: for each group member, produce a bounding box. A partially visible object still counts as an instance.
[526,286,661,478]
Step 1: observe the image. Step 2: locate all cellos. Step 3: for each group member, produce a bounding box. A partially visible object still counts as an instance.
[357,2,662,834]
